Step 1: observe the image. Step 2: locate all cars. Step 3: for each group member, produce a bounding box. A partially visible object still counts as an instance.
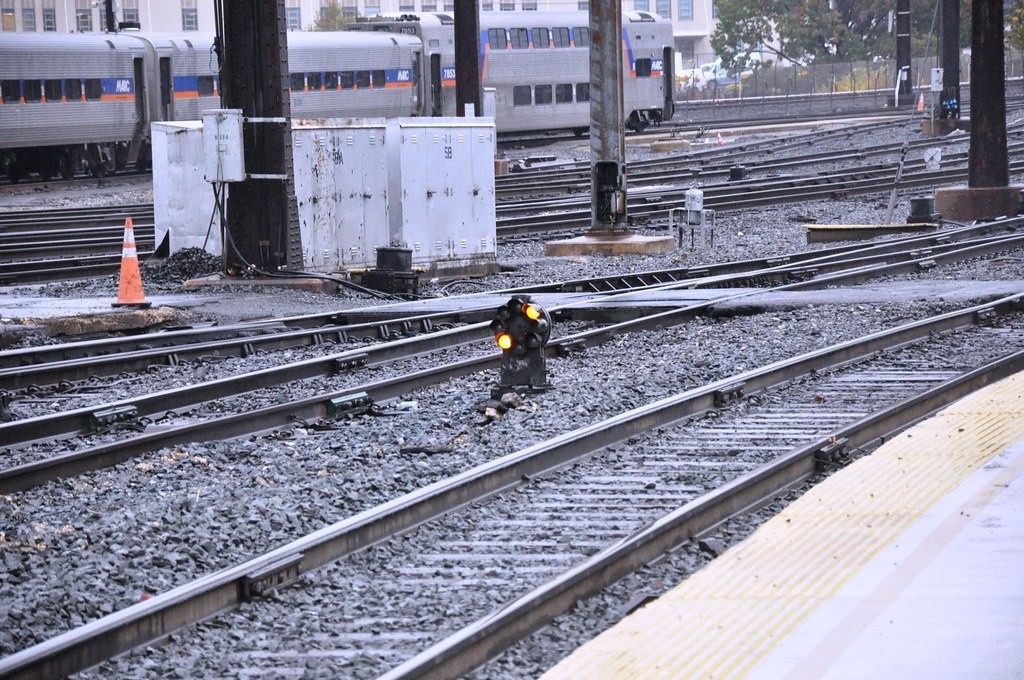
[679,63,711,89]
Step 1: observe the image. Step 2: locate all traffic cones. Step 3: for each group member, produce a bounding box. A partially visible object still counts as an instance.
[110,216,151,309]
[918,94,928,110]
[718,133,725,146]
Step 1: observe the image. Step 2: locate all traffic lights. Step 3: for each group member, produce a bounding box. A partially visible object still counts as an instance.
[491,297,551,356]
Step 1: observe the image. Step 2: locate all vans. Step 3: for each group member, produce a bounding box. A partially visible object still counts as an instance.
[704,52,762,93]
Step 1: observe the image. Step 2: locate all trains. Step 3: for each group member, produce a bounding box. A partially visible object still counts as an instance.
[0,9,677,182]
